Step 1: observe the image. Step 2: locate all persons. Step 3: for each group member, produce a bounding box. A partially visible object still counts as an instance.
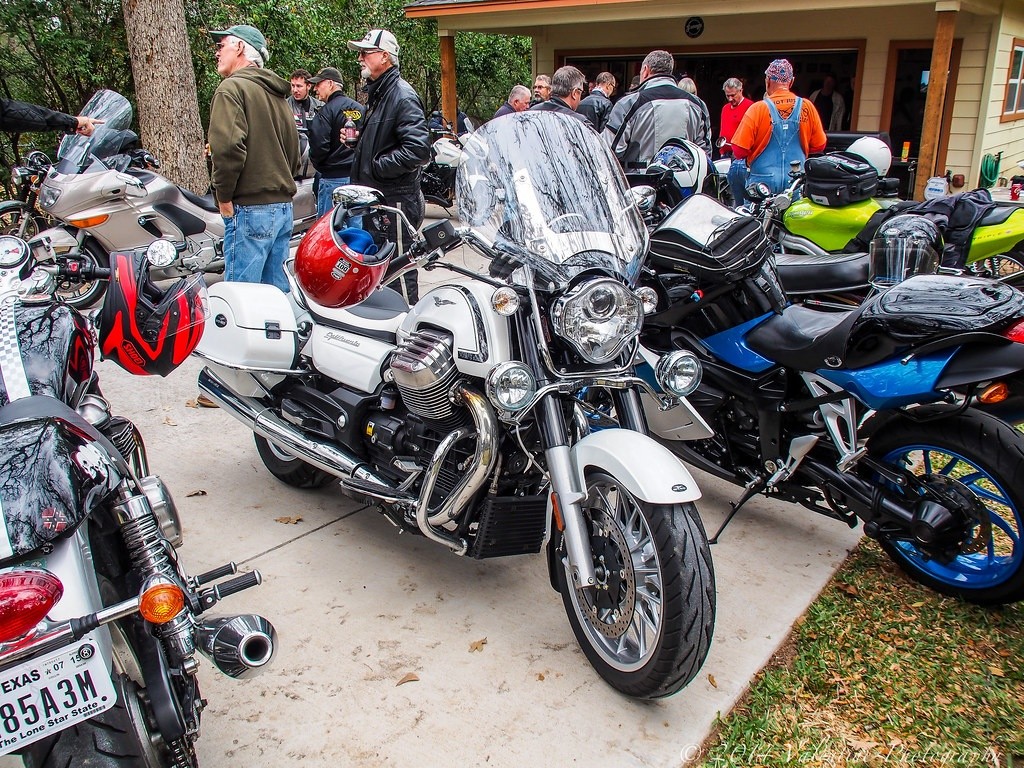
[718,77,754,161]
[731,58,828,208]
[282,68,325,181]
[206,25,302,293]
[307,67,364,232]
[494,71,856,222]
[0,98,106,138]
[340,28,432,307]
[600,50,712,199]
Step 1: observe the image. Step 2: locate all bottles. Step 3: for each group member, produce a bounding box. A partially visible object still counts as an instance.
[344,117,358,148]
[998,176,1007,187]
[901,142,910,162]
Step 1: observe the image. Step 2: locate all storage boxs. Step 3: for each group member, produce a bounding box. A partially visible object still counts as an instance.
[191,281,299,399]
[297,138,317,177]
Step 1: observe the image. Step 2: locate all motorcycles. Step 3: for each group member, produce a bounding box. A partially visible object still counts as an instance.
[13,89,355,310]
[3,187,280,768]
[610,130,1024,606]
[188,110,719,707]
